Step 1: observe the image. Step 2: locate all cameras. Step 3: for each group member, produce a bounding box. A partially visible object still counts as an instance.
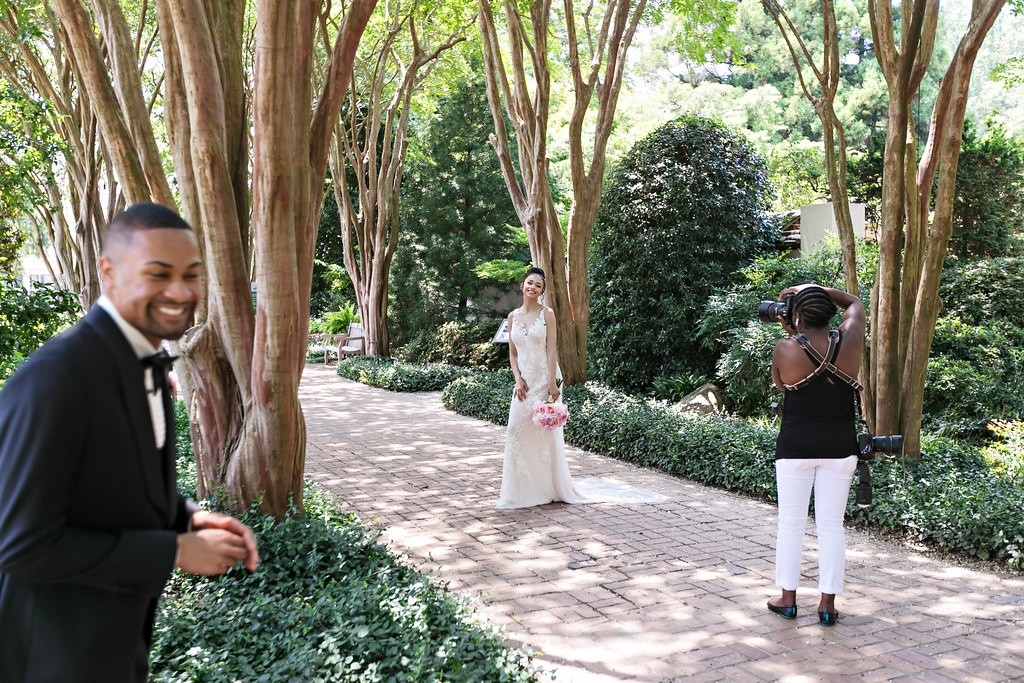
[857,432,903,508]
[758,294,797,327]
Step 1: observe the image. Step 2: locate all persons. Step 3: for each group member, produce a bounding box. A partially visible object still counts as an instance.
[496,267,576,509]
[767,283,866,626]
[0,202,258,683]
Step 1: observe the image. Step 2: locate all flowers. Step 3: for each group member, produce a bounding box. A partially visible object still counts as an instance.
[532,379,570,430]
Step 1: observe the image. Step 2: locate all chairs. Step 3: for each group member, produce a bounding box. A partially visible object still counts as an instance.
[320,320,365,365]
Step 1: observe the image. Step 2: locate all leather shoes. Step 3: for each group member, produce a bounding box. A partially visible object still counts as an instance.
[817,608,838,627]
[767,597,797,619]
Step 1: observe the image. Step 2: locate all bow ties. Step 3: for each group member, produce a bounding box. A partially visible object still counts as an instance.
[139,348,179,395]
